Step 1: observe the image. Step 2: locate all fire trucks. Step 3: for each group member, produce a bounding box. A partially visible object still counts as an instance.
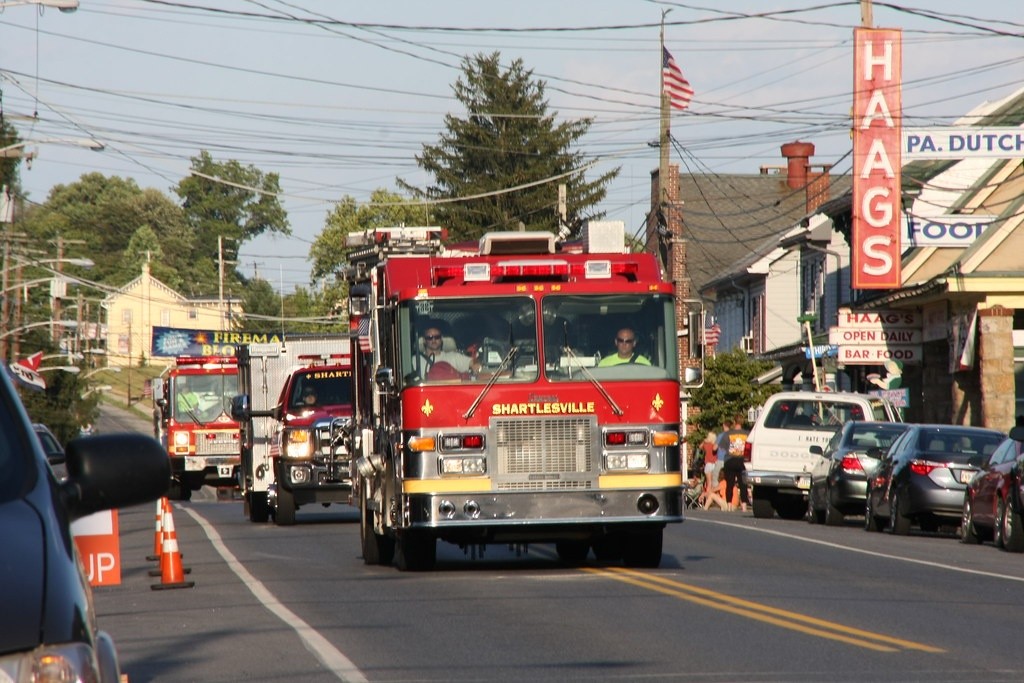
[348,224,700,573]
[230,338,358,527]
[149,355,239,501]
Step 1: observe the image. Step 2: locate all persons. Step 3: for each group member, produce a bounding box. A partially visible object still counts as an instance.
[413,325,480,382]
[177,382,200,419]
[295,386,320,408]
[597,329,652,370]
[698,414,750,512]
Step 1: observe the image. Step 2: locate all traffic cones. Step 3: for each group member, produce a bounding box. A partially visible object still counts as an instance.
[145,496,190,577]
[150,504,194,592]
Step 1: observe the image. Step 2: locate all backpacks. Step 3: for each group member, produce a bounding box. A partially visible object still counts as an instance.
[728,434,747,457]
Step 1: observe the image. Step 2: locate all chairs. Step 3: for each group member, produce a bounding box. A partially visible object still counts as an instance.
[418,337,456,354]
[854,438,876,447]
[928,440,945,452]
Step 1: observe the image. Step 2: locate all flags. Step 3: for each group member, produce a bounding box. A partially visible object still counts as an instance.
[701,311,721,347]
[358,319,375,352]
[663,44,695,111]
[8,352,47,396]
[143,379,152,395]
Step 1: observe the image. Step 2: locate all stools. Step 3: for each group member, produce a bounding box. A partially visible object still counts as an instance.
[683,488,702,510]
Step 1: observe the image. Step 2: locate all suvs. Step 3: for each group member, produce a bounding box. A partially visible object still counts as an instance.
[742,390,903,521]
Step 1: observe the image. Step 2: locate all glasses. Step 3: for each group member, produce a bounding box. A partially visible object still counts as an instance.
[425,334,442,341]
[616,337,634,343]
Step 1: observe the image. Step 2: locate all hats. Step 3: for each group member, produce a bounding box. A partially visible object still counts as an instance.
[304,388,317,396]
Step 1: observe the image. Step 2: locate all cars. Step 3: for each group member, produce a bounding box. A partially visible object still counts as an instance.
[30,421,68,482]
[960,436,1024,554]
[0,362,174,683]
[864,424,1008,539]
[805,419,913,527]
